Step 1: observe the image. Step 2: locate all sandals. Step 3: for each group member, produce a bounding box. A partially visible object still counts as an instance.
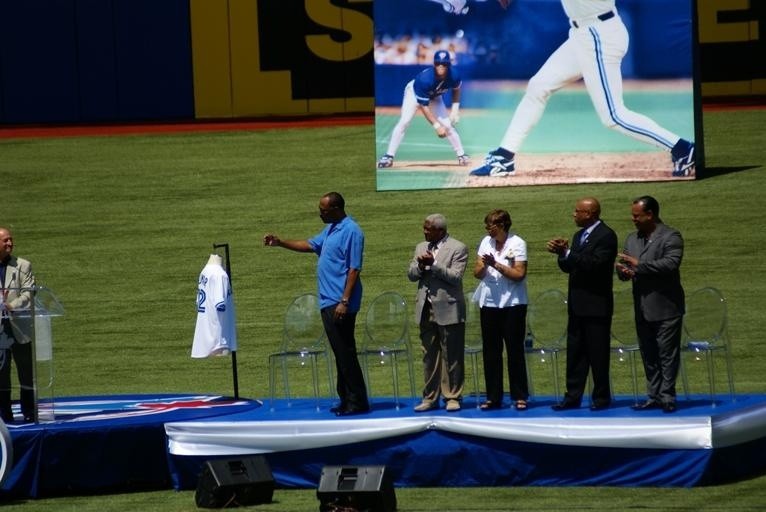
[515,399,527,410]
[480,400,502,410]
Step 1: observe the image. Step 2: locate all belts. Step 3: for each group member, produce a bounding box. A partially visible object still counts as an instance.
[573,11,615,28]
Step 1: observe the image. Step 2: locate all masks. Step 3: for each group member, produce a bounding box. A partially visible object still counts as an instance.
[485,222,506,238]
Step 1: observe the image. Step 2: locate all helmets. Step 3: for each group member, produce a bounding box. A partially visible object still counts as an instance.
[433,50,452,65]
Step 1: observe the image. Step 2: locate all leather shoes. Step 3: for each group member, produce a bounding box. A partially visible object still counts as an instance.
[662,401,676,413]
[551,403,581,411]
[330,405,345,413]
[414,402,441,412]
[445,399,461,411]
[335,404,370,416]
[590,404,599,410]
[631,398,662,410]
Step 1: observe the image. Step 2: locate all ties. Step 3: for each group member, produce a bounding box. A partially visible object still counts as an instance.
[579,230,588,247]
[429,245,436,256]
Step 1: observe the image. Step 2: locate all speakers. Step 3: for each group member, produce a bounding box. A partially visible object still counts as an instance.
[316,465,397,512]
[195,454,274,508]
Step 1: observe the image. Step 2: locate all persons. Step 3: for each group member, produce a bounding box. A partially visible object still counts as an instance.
[478,206,532,412]
[616,194,687,413]
[377,49,473,169]
[374,32,470,67]
[545,196,620,411]
[264,191,374,417]
[405,213,469,412]
[0,226,38,424]
[190,253,237,358]
[433,1,695,178]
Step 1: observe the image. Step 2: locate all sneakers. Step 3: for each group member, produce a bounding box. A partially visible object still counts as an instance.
[670,138,695,178]
[378,154,393,169]
[468,152,517,179]
[458,155,469,167]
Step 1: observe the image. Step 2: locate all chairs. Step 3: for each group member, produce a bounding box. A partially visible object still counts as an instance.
[679,284,738,415]
[352,292,418,412]
[455,287,491,415]
[517,288,576,409]
[602,286,649,409]
[264,292,335,414]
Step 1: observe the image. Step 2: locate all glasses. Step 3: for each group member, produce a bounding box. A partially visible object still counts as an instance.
[572,211,585,217]
